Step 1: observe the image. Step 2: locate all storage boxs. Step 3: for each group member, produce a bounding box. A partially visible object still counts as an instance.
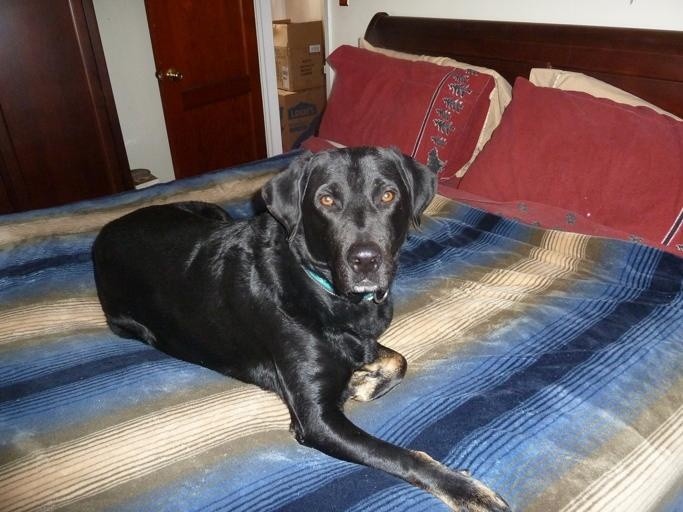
[271,18,327,92]
[276,88,328,154]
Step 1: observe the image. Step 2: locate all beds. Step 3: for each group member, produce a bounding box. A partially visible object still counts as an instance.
[1,10,683,512]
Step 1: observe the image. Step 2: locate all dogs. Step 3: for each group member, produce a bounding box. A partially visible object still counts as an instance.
[89,144,512,512]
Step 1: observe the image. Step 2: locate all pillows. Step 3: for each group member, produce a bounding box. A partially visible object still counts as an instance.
[302,43,491,185]
[356,37,510,176]
[529,68,681,122]
[437,76,683,259]
